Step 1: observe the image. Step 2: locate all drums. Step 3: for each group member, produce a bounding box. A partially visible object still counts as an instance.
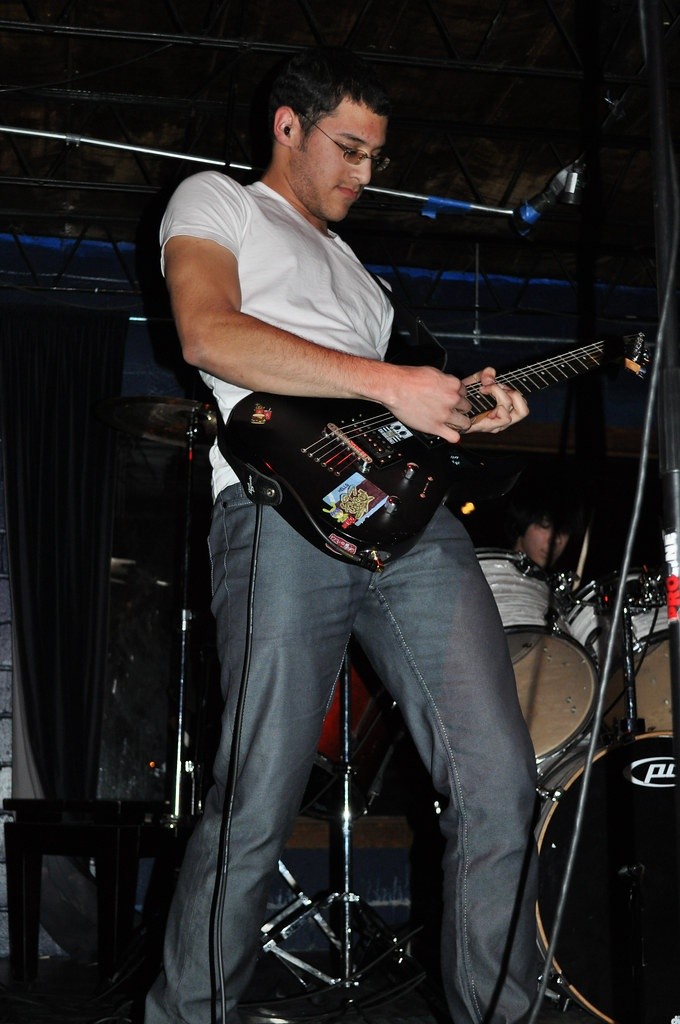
[317,639,384,786]
[532,728,680,1020]
[594,574,674,737]
[472,544,600,764]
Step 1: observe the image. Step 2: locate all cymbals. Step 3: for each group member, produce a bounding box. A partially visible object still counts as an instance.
[102,394,218,451]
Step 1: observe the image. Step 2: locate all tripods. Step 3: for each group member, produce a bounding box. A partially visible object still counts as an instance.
[257,642,454,1024]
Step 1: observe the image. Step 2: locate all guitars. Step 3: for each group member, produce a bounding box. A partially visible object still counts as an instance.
[223,326,659,568]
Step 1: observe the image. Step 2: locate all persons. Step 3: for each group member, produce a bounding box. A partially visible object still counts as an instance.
[146,43,595,1024]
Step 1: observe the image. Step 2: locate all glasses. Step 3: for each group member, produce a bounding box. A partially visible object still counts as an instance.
[295,107,390,172]
